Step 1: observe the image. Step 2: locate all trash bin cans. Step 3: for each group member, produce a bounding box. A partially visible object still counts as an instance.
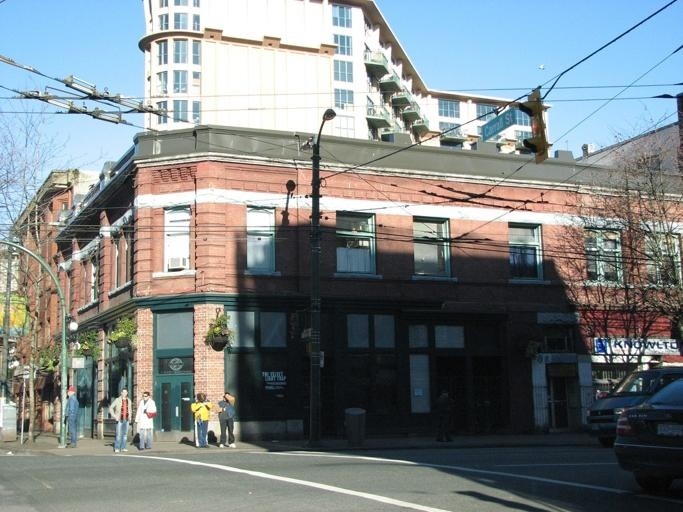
[345,407,367,446]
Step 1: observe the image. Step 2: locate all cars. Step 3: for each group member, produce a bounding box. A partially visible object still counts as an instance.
[615,374,681,500]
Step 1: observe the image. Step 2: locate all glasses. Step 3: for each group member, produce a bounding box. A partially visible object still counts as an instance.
[143,396,148,397]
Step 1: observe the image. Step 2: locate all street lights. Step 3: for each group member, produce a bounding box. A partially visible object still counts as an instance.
[58,314,80,449]
[306,110,337,451]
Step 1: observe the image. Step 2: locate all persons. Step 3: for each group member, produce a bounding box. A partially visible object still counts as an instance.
[215,392,237,448]
[134,392,157,450]
[436,389,456,442]
[191,392,213,448]
[109,388,132,453]
[64,386,79,448]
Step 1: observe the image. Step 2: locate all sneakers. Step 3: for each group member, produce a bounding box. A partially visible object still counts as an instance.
[219,443,236,448]
[66,444,76,448]
[114,448,128,453]
[140,447,151,450]
[200,445,208,448]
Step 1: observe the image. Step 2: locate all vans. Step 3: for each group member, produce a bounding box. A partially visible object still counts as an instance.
[584,366,682,449]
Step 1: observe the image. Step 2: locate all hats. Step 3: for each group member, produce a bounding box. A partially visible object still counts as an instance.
[68,386,76,392]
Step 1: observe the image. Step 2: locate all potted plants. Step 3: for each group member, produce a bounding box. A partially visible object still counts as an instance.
[105,316,138,349]
[202,313,235,357]
[73,335,101,361]
